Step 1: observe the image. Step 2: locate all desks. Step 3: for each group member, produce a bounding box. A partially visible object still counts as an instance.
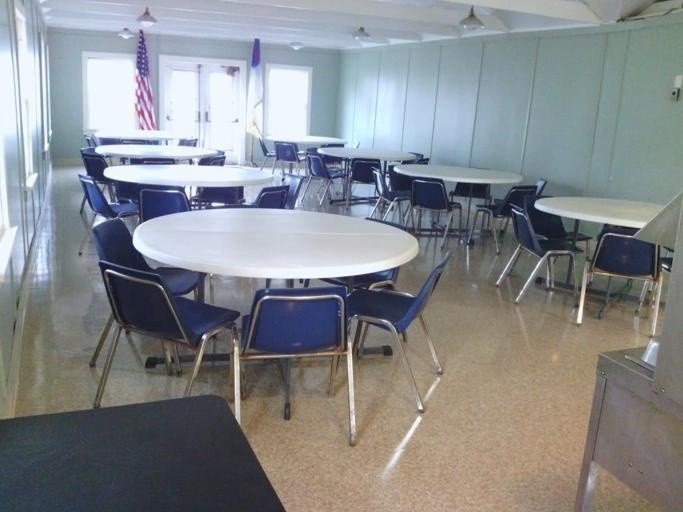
[2,393,289,512]
[132,207,420,420]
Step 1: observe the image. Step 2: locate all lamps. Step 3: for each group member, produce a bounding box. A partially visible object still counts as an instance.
[457,4,487,31]
[135,6,157,27]
[352,27,372,42]
[115,27,135,40]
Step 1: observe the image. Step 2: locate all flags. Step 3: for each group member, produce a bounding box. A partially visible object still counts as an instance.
[242,39,267,142]
[134,30,156,130]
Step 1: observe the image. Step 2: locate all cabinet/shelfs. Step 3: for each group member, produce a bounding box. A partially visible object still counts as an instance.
[569,201,683,510]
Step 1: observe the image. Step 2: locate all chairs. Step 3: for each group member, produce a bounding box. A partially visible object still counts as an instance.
[93,259,244,408]
[316,216,411,355]
[228,289,356,447]
[79,131,430,256]
[369,151,547,255]
[496,194,677,339]
[88,218,207,377]
[332,250,454,412]
